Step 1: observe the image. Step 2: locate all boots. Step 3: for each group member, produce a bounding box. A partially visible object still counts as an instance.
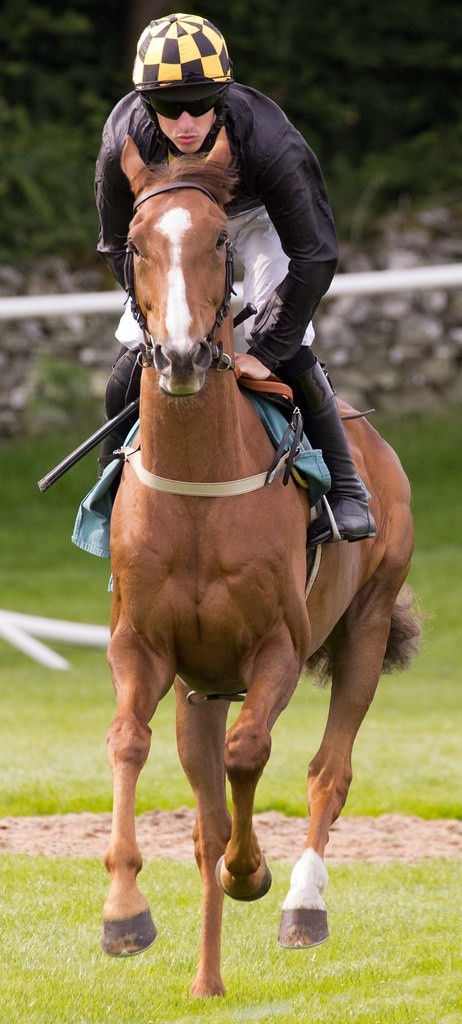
[97,414,124,481]
[283,355,376,543]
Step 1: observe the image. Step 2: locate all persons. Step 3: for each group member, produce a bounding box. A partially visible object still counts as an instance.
[97,14,378,542]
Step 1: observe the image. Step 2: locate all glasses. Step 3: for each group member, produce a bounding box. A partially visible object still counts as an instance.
[149,94,219,120]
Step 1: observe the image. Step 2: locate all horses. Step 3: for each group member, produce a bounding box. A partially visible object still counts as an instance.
[97,126,439,998]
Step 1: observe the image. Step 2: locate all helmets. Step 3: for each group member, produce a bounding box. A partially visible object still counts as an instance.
[132,12,234,101]
